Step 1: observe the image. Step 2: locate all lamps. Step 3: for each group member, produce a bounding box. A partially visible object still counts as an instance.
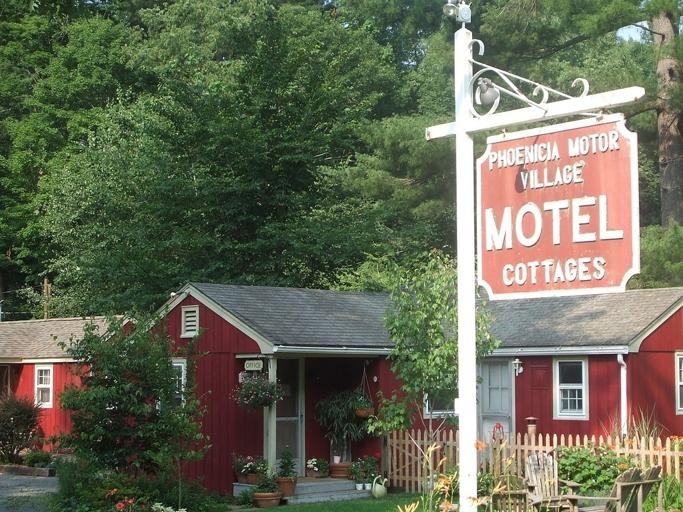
[442,0,458,17]
[511,358,523,376]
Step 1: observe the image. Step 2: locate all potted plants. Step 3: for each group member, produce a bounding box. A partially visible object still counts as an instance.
[232,387,377,507]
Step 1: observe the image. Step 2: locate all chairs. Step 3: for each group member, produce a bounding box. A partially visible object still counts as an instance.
[489,452,663,512]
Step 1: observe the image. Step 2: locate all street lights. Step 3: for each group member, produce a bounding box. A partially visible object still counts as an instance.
[442,0,484,512]
